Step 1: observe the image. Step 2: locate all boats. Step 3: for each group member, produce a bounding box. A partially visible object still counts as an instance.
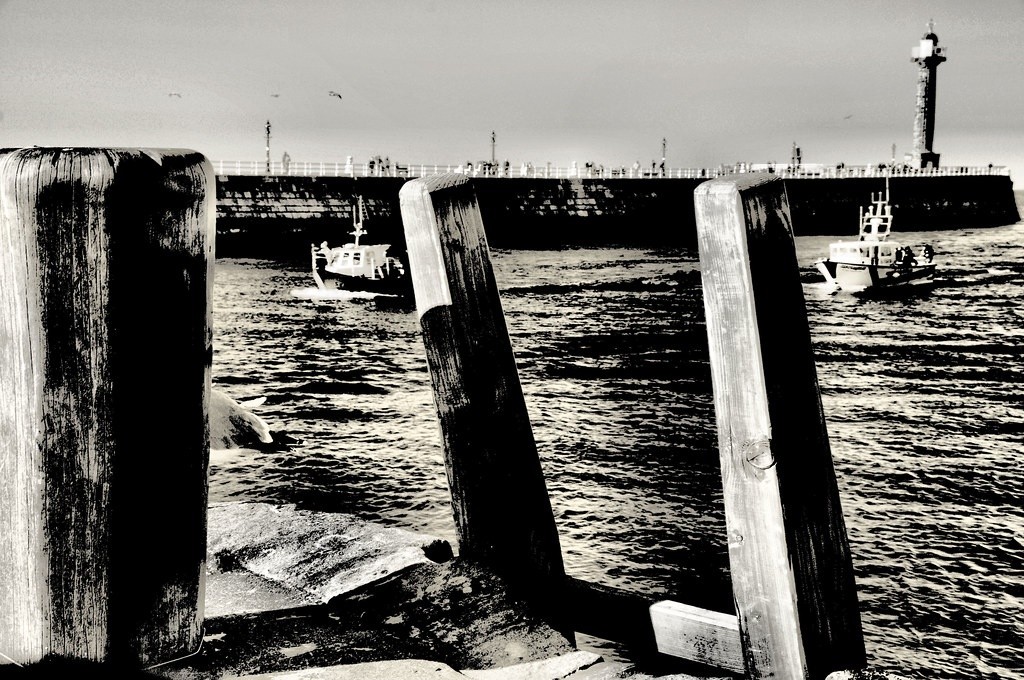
[310,196,415,302]
[809,174,938,299]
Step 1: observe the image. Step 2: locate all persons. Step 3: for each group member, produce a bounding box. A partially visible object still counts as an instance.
[585,158,666,176]
[988,164,993,175]
[894,243,934,264]
[282,152,291,175]
[768,161,775,173]
[467,159,509,177]
[878,163,924,174]
[368,155,409,176]
[836,162,844,175]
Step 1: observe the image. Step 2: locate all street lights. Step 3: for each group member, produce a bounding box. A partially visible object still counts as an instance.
[490,130,495,177]
[662,137,665,177]
[791,142,797,176]
[264,120,272,175]
